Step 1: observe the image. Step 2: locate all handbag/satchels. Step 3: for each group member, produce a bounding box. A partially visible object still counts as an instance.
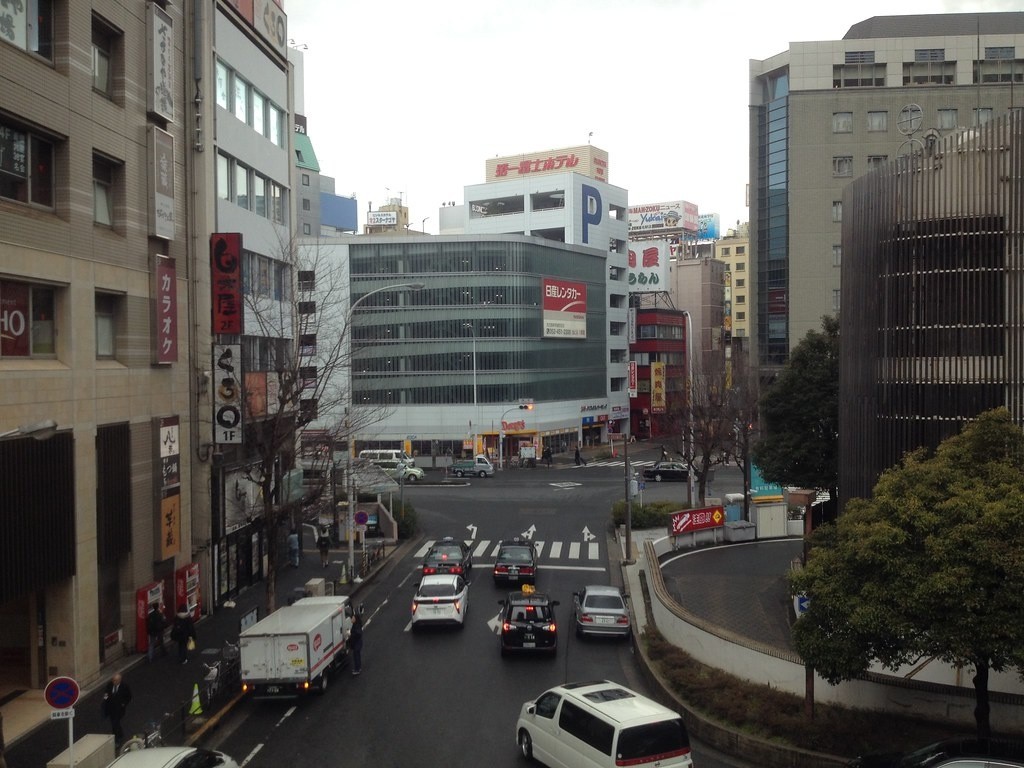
[101,700,110,718]
[187,639,196,650]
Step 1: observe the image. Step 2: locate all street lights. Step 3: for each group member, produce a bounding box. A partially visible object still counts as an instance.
[683,310,696,508]
[347,281,426,581]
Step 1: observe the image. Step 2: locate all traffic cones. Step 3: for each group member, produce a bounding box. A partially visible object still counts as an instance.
[339,564,349,584]
[188,683,203,715]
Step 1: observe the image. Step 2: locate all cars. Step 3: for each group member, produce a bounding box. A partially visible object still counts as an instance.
[494,540,538,588]
[368,459,425,482]
[423,539,473,580]
[643,459,702,483]
[574,584,631,643]
[411,574,469,633]
[104,746,239,768]
[495,584,561,660]
[843,730,1024,768]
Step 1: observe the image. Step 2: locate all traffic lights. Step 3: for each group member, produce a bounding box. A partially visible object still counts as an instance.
[519,404,532,409]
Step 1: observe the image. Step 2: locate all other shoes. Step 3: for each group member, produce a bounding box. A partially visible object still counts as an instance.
[182,659,192,664]
[352,672,360,675]
[352,669,362,672]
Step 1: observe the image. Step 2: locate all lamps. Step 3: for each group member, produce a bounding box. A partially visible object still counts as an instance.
[287,39,308,49]
[0,419,58,441]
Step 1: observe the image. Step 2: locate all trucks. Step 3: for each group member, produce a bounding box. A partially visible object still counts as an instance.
[450,454,497,479]
[238,593,366,706]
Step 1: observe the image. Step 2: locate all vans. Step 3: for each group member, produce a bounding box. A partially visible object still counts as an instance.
[515,678,694,768]
[358,449,415,468]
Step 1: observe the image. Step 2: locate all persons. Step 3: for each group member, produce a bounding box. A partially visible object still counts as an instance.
[171,605,196,664]
[574,446,580,465]
[661,445,667,461]
[631,431,636,444]
[317,528,331,567]
[101,674,131,748]
[544,446,552,467]
[287,529,299,568]
[346,615,363,676]
[147,602,167,653]
